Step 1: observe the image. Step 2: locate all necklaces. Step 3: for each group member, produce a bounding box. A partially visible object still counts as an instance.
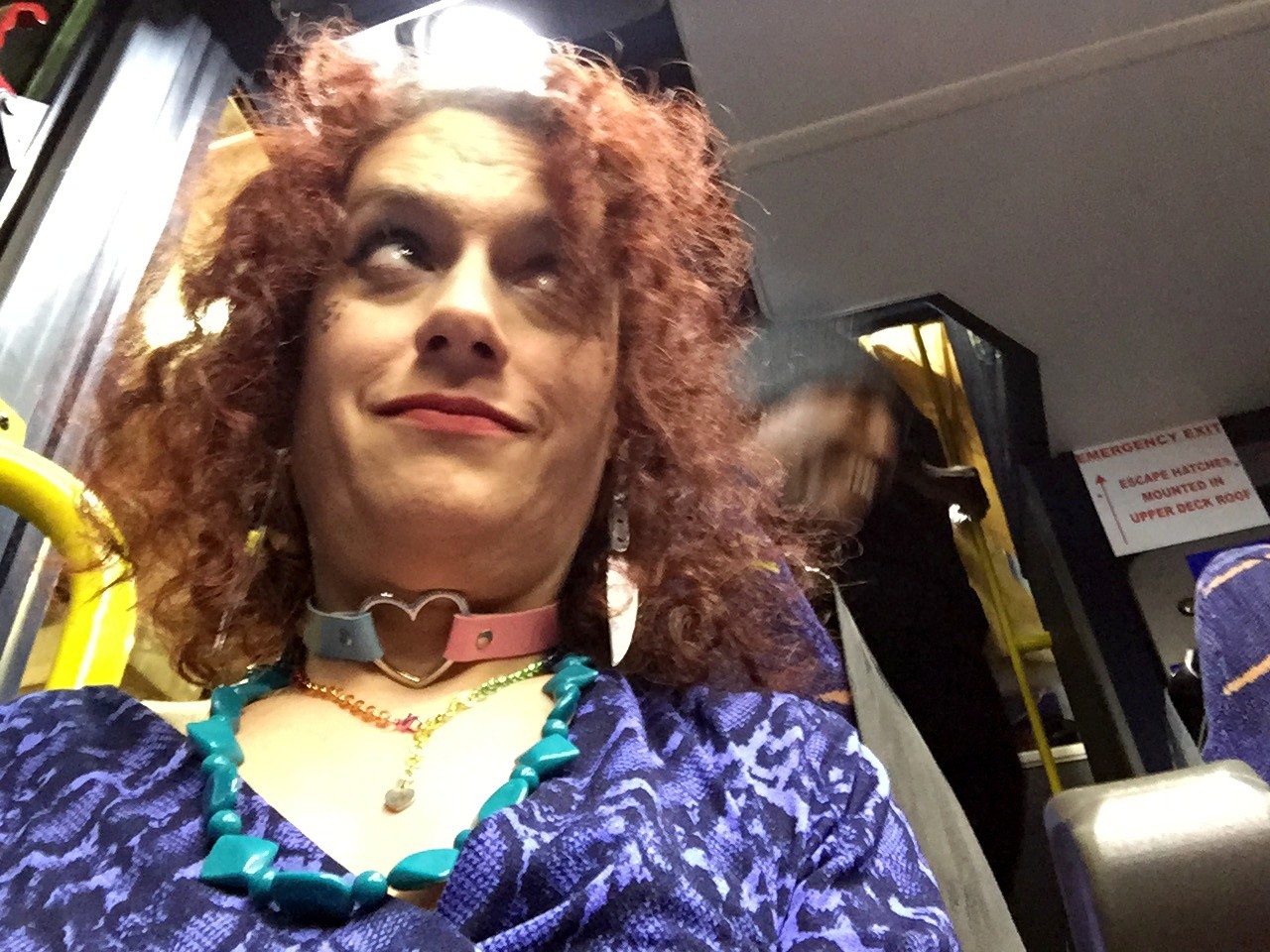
[298,588,560,691]
[282,654,561,814]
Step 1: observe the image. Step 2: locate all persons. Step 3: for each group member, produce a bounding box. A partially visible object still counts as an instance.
[1,0,1017,950]
